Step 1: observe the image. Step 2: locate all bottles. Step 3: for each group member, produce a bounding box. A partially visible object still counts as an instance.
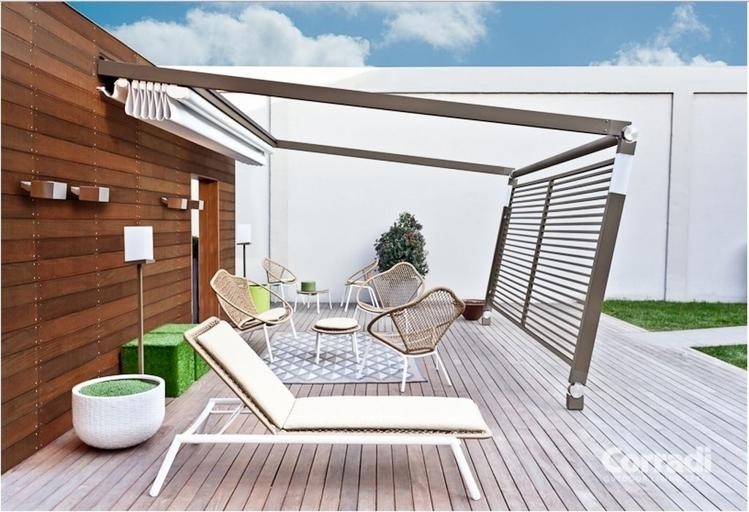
[481,298,491,326]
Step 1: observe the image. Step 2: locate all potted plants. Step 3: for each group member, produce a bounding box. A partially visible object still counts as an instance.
[373,213,430,307]
[71,373,167,450]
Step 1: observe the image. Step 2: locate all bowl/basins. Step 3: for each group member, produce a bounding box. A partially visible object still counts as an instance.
[451,299,488,320]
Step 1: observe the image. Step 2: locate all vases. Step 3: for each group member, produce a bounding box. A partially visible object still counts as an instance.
[461,298,487,320]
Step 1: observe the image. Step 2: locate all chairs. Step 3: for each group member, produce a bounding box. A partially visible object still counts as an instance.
[148,314,493,501]
[210,257,467,393]
